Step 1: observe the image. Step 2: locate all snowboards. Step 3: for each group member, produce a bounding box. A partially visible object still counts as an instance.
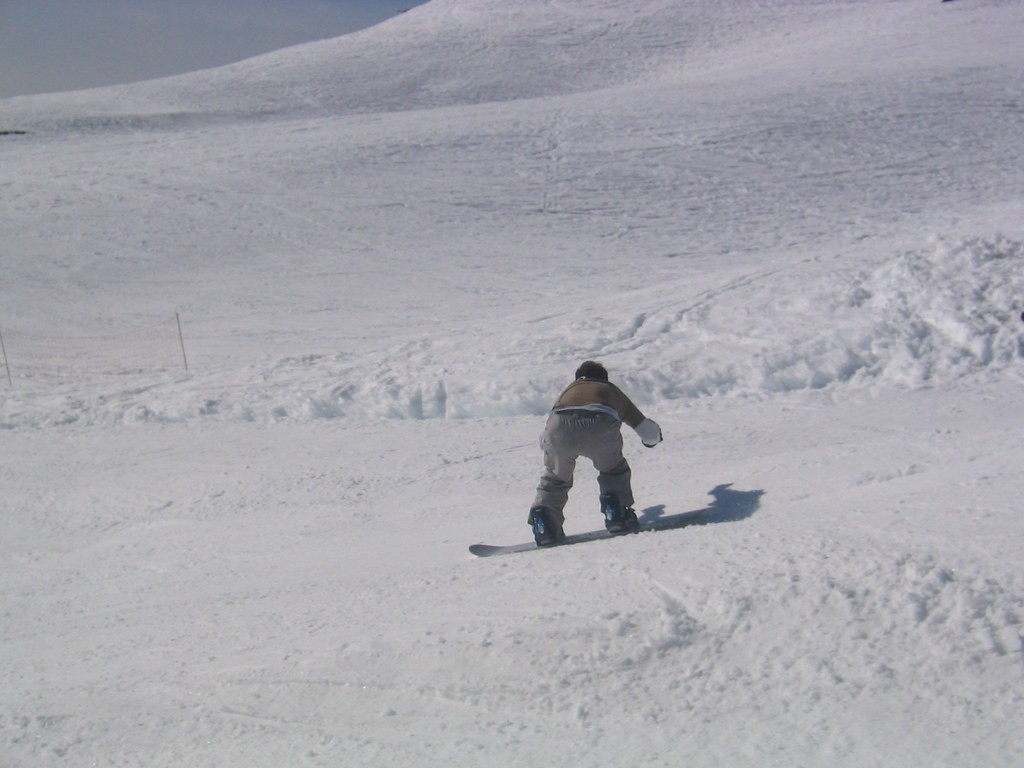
[468,517,652,558]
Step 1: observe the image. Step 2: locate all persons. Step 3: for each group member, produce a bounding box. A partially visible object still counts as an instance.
[527,360,663,547]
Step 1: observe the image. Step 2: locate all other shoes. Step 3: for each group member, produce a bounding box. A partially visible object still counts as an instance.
[531,508,556,547]
[602,493,637,533]
